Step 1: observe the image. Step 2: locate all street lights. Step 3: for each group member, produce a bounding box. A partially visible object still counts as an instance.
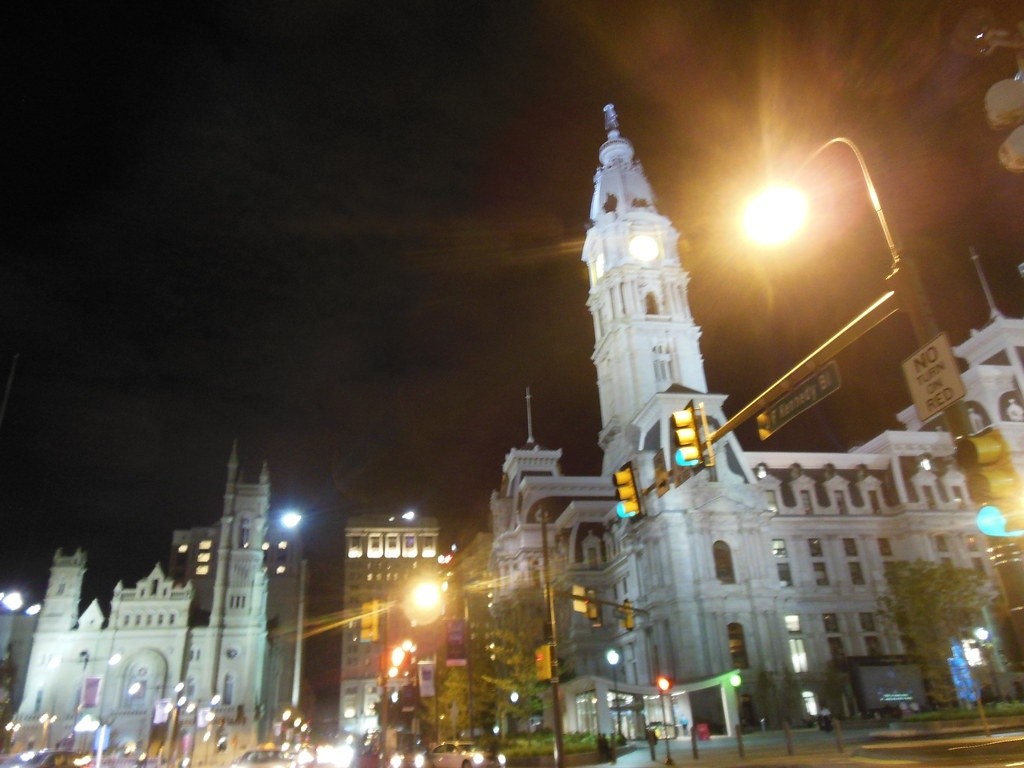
[740,133,1024,666]
[605,648,622,736]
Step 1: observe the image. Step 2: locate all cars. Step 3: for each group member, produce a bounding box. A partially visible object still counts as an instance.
[0,737,508,768]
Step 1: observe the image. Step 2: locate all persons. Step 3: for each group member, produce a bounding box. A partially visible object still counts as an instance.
[603,104,619,131]
[820,704,832,732]
[596,733,610,762]
[856,699,919,720]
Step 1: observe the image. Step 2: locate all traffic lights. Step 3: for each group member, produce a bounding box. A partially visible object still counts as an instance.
[956,429,1024,539]
[359,602,373,643]
[657,675,674,692]
[612,461,643,520]
[673,399,707,465]
[535,645,551,682]
